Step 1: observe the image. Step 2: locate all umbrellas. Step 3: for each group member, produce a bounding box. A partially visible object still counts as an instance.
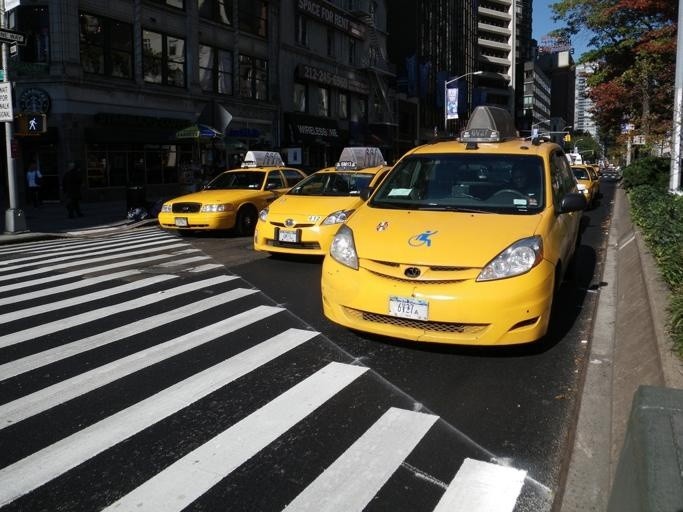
[169,122,224,177]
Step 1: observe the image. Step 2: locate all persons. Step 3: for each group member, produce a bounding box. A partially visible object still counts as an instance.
[26,162,47,211]
[506,165,538,203]
[61,162,84,219]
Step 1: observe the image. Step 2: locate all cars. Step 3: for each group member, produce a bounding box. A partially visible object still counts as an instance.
[157,151,307,236]
[253,147,393,255]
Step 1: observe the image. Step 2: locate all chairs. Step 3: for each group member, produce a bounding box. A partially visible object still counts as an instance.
[426,163,455,199]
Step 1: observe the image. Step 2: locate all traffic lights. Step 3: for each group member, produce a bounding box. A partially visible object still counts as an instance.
[25,114,43,133]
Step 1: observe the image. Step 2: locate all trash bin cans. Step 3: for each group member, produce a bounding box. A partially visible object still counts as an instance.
[125,182,146,211]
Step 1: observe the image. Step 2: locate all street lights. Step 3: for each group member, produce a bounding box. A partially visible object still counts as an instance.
[444,70,484,128]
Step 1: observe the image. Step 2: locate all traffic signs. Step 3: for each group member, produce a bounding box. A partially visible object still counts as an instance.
[0,28,26,44]
